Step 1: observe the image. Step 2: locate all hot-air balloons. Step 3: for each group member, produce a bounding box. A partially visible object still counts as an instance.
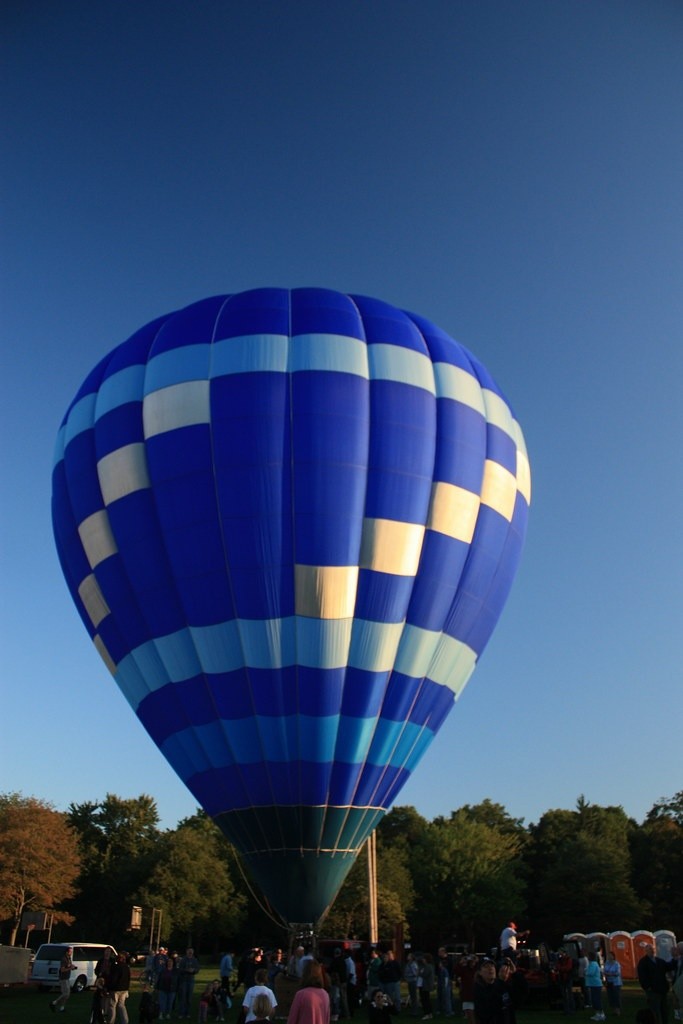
[49,286,533,936]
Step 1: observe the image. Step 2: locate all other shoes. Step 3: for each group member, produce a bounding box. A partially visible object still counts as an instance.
[215,1016,225,1022]
[421,1013,433,1020]
[178,1015,185,1019]
[186,1015,192,1019]
[48,1001,56,1013]
[347,1016,352,1021]
[158,1014,164,1020]
[330,1015,339,1021]
[166,1014,171,1019]
[58,1008,70,1017]
[584,1004,592,1008]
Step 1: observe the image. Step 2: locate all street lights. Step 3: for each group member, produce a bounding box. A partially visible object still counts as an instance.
[131,906,164,954]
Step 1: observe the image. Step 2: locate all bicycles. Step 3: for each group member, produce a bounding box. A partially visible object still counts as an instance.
[139,971,160,988]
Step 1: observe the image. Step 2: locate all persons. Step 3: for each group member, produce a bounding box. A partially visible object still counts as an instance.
[145,921,621,1024]
[637,942,683,1024]
[89,946,131,1024]
[49,947,77,1015]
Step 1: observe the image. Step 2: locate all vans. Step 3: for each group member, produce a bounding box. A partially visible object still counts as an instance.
[28,945,120,997]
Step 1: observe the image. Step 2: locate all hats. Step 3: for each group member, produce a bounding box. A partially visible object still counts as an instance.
[478,956,496,966]
[508,922,516,928]
[296,946,304,951]
[334,947,342,954]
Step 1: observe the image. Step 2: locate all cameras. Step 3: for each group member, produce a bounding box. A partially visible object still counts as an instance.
[503,959,510,966]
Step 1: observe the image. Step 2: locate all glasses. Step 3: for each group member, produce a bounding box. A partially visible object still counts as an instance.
[377,996,384,999]
[502,963,510,966]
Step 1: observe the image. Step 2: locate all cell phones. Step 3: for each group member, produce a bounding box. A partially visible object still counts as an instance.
[383,994,387,1000]
